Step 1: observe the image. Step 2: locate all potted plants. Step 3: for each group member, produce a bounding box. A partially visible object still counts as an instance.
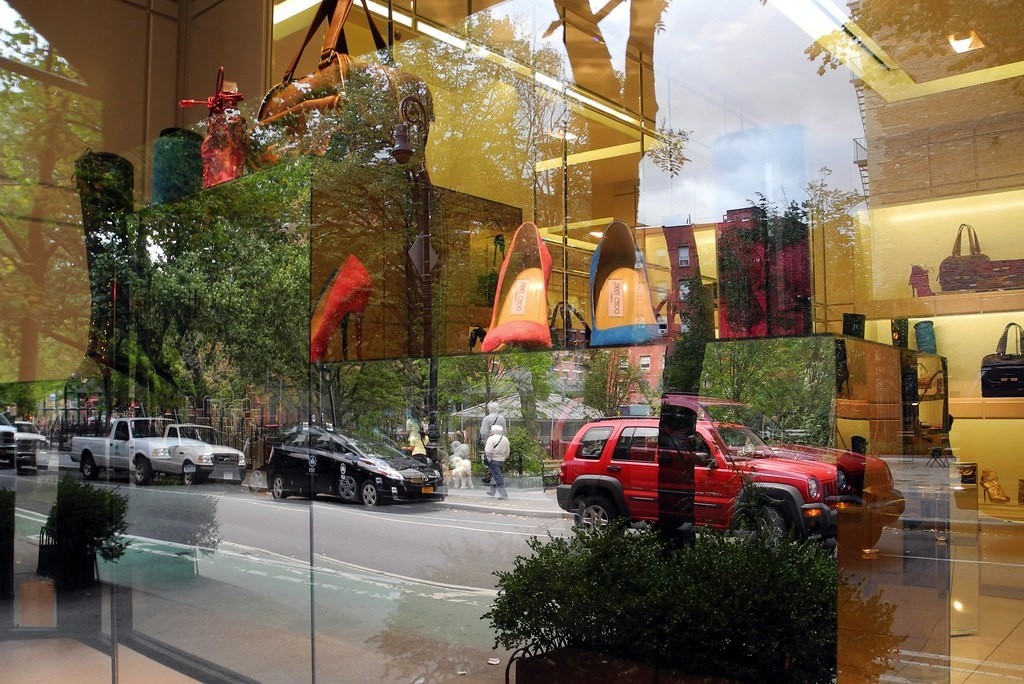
[472,512,906,684]
[43,478,130,632]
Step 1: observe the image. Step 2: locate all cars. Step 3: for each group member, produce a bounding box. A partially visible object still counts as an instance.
[250,425,449,504]
[78,414,116,429]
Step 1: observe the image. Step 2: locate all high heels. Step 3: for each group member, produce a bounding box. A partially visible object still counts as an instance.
[590,220,663,346]
[321,269,349,361]
[481,222,552,353]
[908,264,936,296]
[980,471,1010,503]
[309,254,372,362]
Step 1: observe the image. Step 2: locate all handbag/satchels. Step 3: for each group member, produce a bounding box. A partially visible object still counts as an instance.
[981,322,1024,398]
[244,0,435,173]
[550,300,591,349]
[484,454,489,466]
[916,362,932,389]
[179,67,250,189]
[976,258,1024,293]
[936,223,991,291]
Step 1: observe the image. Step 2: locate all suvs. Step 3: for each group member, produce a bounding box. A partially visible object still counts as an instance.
[546,392,790,444]
[556,417,906,556]
[13,420,51,470]
[0,413,14,468]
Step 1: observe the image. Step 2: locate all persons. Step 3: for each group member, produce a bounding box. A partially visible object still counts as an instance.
[401,423,429,463]
[480,402,507,487]
[485,425,510,500]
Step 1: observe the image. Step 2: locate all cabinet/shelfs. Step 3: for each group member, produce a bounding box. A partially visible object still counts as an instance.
[864,289,1024,420]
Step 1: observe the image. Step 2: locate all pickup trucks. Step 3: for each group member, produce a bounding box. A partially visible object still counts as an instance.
[148,422,247,487]
[70,417,216,485]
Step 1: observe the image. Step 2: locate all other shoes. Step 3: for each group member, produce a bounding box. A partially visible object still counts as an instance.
[487,492,495,496]
[482,478,490,483]
[498,497,508,500]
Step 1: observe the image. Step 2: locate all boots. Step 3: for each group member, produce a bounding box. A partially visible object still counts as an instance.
[835,338,849,394]
[150,137,203,204]
[70,147,154,393]
